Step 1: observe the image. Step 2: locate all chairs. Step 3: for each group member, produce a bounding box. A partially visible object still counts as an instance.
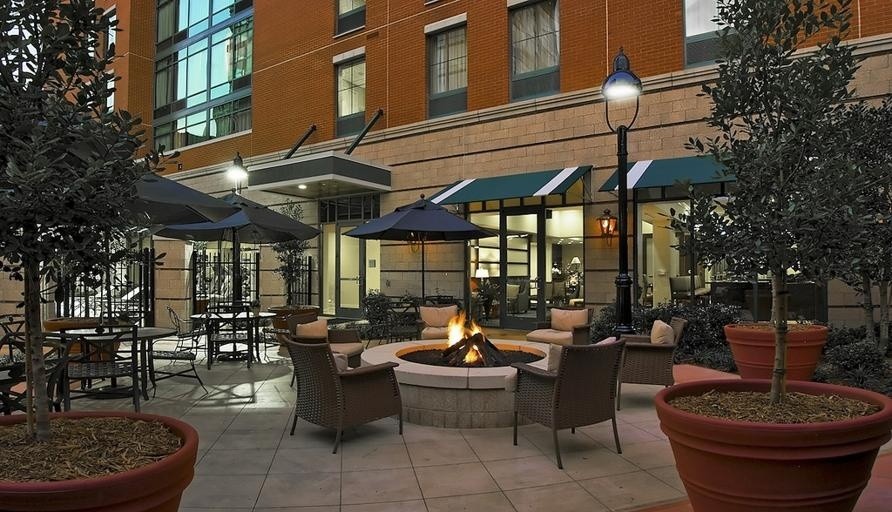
[525,302,598,350]
[529,271,581,304]
[285,310,367,387]
[500,277,532,316]
[1,299,296,417]
[610,313,688,412]
[508,336,629,470]
[361,291,470,350]
[280,329,407,454]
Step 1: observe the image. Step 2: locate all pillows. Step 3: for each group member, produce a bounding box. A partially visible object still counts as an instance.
[547,304,590,333]
[546,337,617,376]
[332,351,350,375]
[505,284,521,299]
[648,319,675,346]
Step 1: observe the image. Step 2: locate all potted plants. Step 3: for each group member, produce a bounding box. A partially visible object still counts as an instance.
[1,0,204,511]
[266,197,324,343]
[654,1,891,512]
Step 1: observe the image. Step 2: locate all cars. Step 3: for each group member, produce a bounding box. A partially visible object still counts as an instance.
[53,279,151,329]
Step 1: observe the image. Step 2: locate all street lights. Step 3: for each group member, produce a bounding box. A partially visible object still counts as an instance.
[224,150,249,313]
[599,44,649,337]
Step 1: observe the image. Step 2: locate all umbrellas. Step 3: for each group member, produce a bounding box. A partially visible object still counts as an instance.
[337,193,501,305]
[152,189,325,364]
[82,164,242,400]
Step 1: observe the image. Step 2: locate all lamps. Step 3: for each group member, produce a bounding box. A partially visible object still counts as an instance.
[475,266,491,290]
[593,205,625,245]
